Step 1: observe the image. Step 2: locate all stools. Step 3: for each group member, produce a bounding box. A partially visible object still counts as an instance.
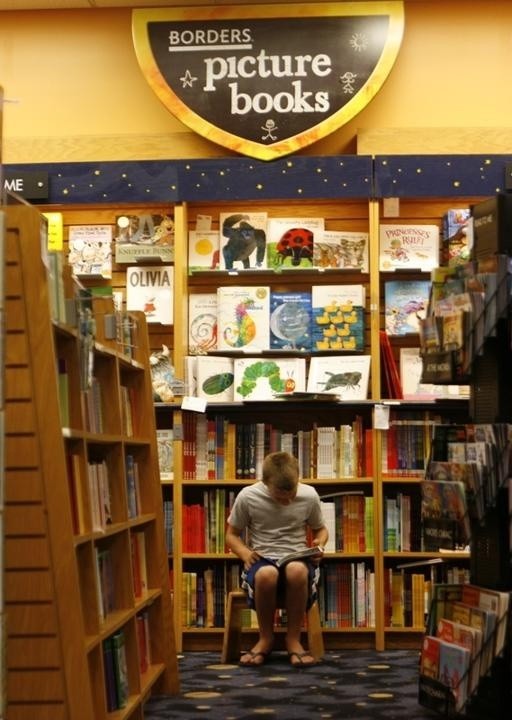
[216,585,325,664]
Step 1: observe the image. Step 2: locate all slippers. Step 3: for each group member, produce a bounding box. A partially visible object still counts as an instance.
[236,648,273,667]
[287,651,319,668]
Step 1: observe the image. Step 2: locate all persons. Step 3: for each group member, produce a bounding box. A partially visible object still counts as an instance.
[223,451,331,670]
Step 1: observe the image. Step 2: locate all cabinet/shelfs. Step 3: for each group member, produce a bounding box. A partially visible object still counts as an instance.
[135,403,177,646]
[374,212,467,643]
[1,198,180,717]
[178,197,379,646]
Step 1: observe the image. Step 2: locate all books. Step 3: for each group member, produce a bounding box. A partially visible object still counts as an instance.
[450,600,493,677]
[436,617,485,696]
[462,584,512,658]
[252,543,323,569]
[421,636,469,717]
